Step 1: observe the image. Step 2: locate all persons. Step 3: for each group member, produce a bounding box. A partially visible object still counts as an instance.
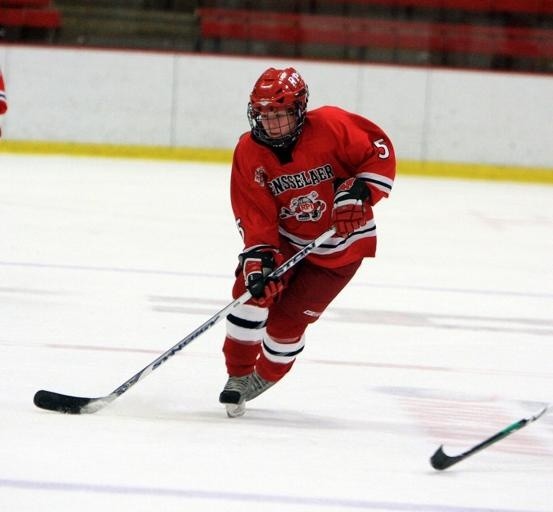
[219,67,396,404]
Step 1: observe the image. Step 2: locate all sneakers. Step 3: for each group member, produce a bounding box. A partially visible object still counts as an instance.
[219,370,275,405]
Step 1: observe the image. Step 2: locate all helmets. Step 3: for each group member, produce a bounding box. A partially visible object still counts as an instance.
[250,68,309,119]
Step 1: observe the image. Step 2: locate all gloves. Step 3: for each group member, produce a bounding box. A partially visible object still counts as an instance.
[243,248,290,304]
[331,178,371,237]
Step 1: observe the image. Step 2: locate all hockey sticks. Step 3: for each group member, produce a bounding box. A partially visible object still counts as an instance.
[34,206,367,413]
[429,404,551,470]
